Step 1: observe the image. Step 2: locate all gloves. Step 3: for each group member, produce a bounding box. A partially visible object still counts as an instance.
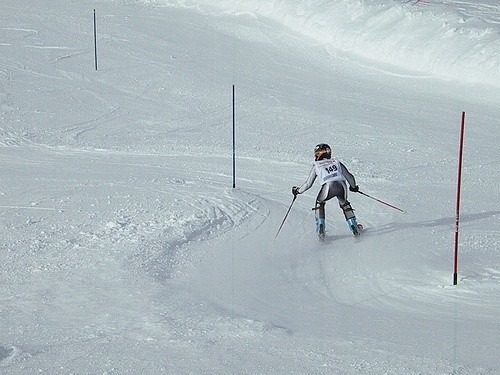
[292,187,298,195]
[350,186,359,192]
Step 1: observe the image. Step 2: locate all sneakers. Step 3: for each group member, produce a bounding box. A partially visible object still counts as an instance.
[352,224,363,237]
[318,231,326,241]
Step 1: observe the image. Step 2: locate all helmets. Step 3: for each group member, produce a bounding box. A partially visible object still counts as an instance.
[314,144,331,161]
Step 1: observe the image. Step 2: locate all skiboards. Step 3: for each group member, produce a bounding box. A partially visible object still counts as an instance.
[316,223,365,248]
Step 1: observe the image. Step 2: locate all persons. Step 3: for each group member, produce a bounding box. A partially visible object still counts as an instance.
[292,143,363,240]
